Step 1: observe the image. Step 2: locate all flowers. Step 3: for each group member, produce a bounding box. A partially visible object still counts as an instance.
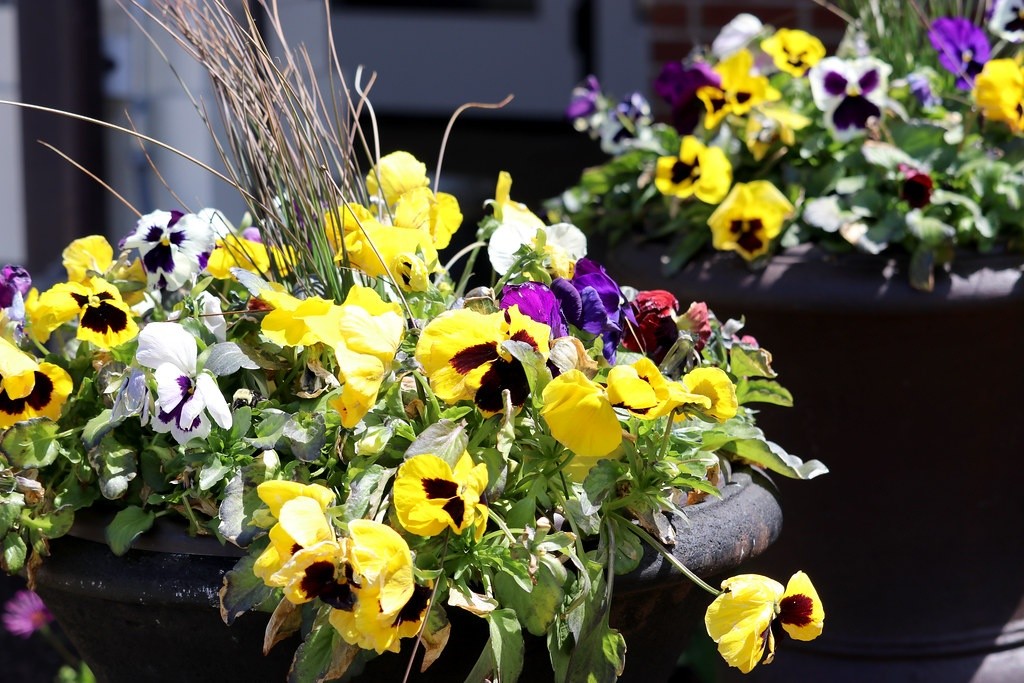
[0,148,829,683]
[541,0,1024,289]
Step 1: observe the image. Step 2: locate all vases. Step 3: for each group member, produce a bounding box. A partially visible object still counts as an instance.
[0,472,785,683]
[606,224,1024,658]
